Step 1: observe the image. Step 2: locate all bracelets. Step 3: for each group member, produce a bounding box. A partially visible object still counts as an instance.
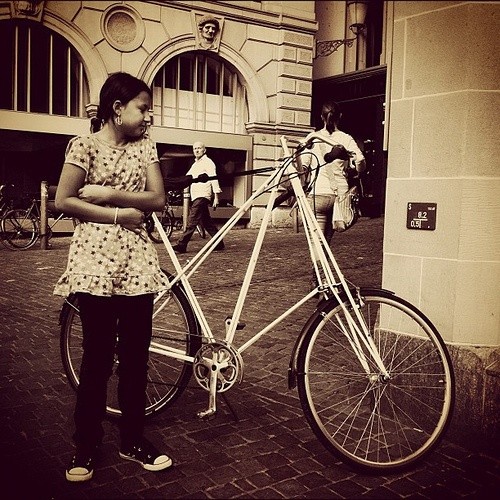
[113,207,119,225]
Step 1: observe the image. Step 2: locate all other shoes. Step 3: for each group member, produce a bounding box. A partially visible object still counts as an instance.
[213,241,225,251]
[311,272,318,292]
[171,243,186,252]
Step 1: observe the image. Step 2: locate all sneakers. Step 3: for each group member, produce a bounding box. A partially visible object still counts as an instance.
[119,436,172,471]
[65,443,99,481]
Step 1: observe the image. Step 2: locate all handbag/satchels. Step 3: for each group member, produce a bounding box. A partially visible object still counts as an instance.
[331,186,359,232]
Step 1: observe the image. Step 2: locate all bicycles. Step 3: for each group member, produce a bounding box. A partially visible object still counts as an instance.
[146,188,206,244]
[0,181,85,252]
[58,132,455,475]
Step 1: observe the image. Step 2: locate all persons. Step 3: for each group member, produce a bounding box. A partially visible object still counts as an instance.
[171,141,225,253]
[52,71,174,483]
[295,100,366,293]
